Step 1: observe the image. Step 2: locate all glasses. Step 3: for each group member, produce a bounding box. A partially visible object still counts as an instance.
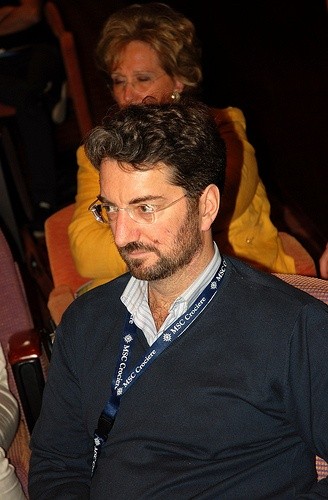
[106,72,168,94]
[88,194,188,224]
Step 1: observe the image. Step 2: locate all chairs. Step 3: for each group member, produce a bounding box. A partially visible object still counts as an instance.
[0,227,56,500]
[45,200,320,329]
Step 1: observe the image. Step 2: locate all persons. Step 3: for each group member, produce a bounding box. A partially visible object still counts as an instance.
[25,96,328,500]
[0,345,26,500]
[67,14,298,294]
